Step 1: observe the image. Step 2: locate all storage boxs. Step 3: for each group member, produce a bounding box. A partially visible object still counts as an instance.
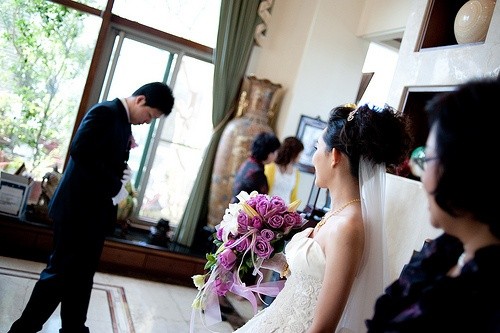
[0,163,35,218]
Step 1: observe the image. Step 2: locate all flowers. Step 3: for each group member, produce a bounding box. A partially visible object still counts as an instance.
[191,190,309,311]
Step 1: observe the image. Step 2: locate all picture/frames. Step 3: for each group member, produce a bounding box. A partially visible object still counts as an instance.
[294,114,327,175]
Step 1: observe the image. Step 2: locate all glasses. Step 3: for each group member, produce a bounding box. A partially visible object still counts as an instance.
[415,156,439,170]
